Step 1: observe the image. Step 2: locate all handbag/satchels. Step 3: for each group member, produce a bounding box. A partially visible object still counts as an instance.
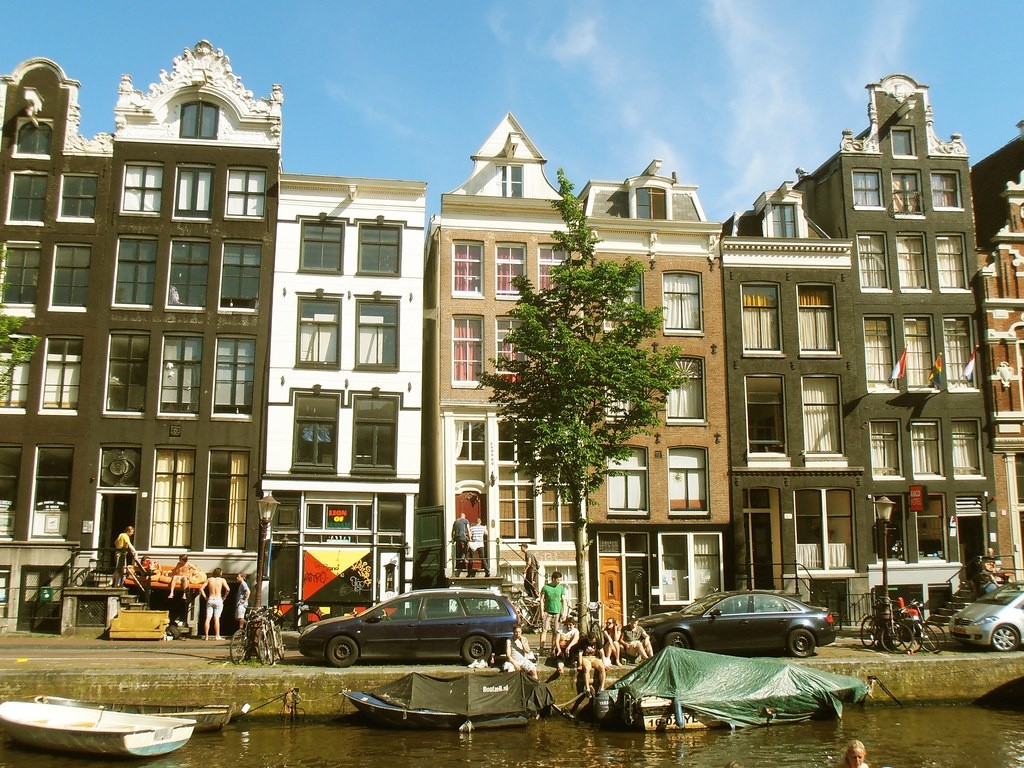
[524,650,535,659]
[535,562,540,570]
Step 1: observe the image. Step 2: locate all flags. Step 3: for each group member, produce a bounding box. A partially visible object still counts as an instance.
[888,349,906,383]
[929,357,941,386]
[962,349,975,381]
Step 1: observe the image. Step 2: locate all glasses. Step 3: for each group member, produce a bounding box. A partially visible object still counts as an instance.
[606,622,613,625]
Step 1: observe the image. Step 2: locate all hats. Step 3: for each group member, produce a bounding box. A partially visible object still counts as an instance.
[630,614,638,621]
[519,543,527,546]
[239,572,247,580]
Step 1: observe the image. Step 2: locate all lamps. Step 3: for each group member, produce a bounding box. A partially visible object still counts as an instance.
[490,471,497,487]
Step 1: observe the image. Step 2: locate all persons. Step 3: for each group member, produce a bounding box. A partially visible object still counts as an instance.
[539,572,653,698]
[138,556,160,610]
[235,572,250,630]
[519,543,540,603]
[112,526,137,588]
[199,568,230,640]
[168,554,197,599]
[502,624,537,679]
[451,512,490,577]
[971,548,1001,596]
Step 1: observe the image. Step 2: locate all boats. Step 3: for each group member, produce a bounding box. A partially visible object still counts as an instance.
[31,694,237,733]
[0,701,197,759]
[591,644,877,734]
[341,668,555,732]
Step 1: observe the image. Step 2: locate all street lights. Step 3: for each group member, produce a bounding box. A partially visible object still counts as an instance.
[254,491,281,607]
[872,493,896,613]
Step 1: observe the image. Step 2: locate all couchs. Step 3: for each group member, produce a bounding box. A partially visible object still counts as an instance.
[109,609,170,641]
[124,564,204,588]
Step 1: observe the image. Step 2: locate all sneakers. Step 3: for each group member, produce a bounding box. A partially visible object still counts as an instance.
[468,660,479,669]
[475,659,488,668]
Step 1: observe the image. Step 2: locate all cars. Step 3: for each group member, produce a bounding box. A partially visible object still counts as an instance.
[949,580,1024,652]
[628,590,837,654]
[297,587,521,669]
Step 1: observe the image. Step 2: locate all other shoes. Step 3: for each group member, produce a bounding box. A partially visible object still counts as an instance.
[215,636,226,641]
[536,598,540,601]
[586,689,590,698]
[525,598,532,601]
[485,572,490,577]
[466,575,471,577]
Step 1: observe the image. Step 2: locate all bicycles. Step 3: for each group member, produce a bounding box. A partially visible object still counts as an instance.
[575,601,602,646]
[860,596,947,654]
[273,591,322,635]
[229,605,286,666]
[565,604,580,629]
[511,598,554,634]
